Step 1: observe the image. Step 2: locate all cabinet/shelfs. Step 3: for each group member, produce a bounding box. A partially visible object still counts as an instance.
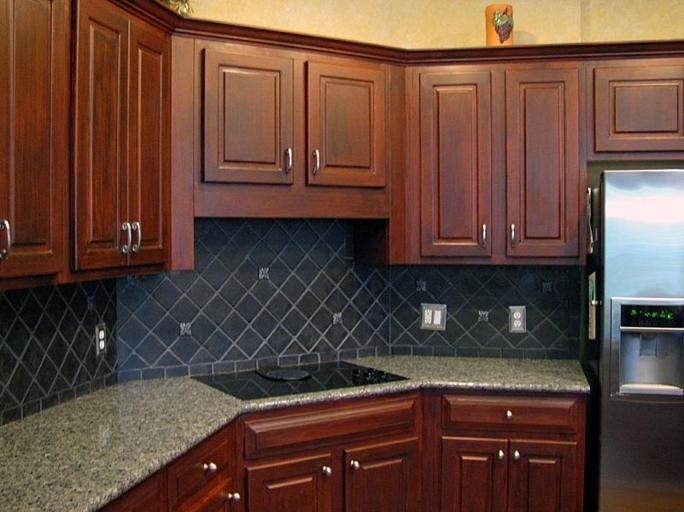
[199,46,394,192]
[242,436,424,509]
[162,424,241,509]
[413,71,587,265]
[432,391,585,512]
[74,0,175,272]
[592,61,684,157]
[0,0,74,281]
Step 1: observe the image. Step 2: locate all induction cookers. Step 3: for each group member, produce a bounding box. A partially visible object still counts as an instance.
[182,360,408,401]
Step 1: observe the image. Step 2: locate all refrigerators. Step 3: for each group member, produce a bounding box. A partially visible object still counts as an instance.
[588,166,683,512]
